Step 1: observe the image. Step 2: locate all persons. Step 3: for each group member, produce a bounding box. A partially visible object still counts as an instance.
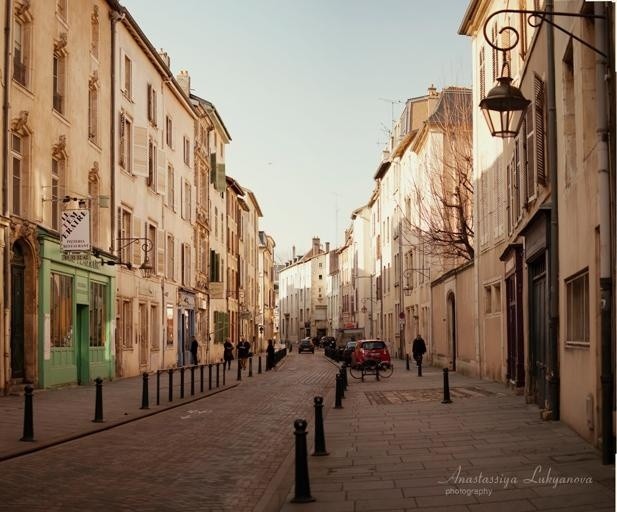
[316,331,321,347]
[330,337,336,349]
[237,337,250,369]
[413,334,426,364]
[223,338,234,369]
[266,339,274,370]
[190,336,198,366]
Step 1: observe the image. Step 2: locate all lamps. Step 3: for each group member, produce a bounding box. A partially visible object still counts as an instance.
[476,9,608,138]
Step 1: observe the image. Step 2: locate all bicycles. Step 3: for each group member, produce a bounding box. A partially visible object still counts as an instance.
[349,357,394,380]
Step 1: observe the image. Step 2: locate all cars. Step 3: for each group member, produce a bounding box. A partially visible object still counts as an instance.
[306,336,336,349]
[350,340,390,371]
[298,338,314,354]
[342,341,357,364]
